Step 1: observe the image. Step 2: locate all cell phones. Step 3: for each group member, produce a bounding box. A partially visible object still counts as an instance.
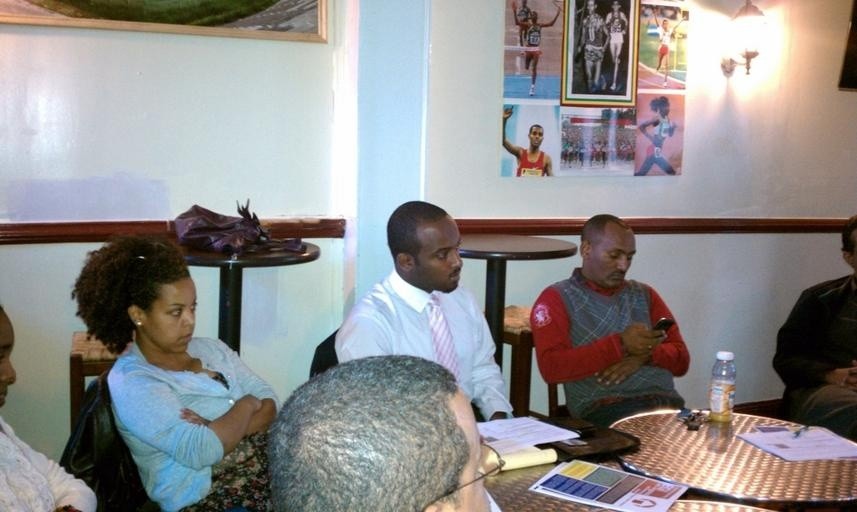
[652,317,675,333]
[555,417,596,438]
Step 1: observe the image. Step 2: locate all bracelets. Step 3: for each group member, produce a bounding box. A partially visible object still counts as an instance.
[618,335,629,357]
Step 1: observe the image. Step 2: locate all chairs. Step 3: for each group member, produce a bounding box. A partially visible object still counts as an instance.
[520,330,569,419]
[307,326,486,423]
[101,380,145,510]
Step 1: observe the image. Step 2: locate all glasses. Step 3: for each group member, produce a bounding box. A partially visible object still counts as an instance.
[421,444,506,512]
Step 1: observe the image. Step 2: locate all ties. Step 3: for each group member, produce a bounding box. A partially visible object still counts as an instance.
[425,294,461,384]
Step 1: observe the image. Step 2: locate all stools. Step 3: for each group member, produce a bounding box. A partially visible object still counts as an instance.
[69,330,119,438]
[503,304,535,416]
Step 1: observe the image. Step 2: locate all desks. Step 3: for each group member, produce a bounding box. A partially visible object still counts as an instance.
[607,409,857,505]
[592,500,778,511]
[156,235,320,358]
[459,232,578,372]
[484,457,624,511]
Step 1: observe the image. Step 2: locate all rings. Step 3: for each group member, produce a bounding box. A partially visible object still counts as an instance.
[647,344,653,350]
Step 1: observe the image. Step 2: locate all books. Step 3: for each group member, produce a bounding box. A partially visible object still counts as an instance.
[488,436,558,471]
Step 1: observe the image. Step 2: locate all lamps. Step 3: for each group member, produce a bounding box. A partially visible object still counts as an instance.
[721,0,764,78]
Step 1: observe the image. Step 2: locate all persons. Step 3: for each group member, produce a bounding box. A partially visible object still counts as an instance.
[577,1,611,91]
[265,353,505,512]
[335,200,515,421]
[70,230,281,512]
[605,0,628,89]
[0,304,98,512]
[772,215,857,445]
[511,0,562,96]
[517,0,532,54]
[530,215,690,428]
[636,96,677,175]
[502,106,554,177]
[650,8,686,87]
[561,123,636,169]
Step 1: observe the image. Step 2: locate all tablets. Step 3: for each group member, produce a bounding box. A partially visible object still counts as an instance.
[534,428,640,465]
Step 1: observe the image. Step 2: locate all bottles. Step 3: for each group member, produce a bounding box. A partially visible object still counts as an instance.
[709,352,736,423]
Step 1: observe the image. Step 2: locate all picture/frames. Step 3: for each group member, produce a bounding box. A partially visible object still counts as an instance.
[1,1,330,45]
[559,0,641,109]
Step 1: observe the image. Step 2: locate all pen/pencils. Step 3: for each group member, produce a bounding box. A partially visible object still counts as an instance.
[792,424,808,439]
